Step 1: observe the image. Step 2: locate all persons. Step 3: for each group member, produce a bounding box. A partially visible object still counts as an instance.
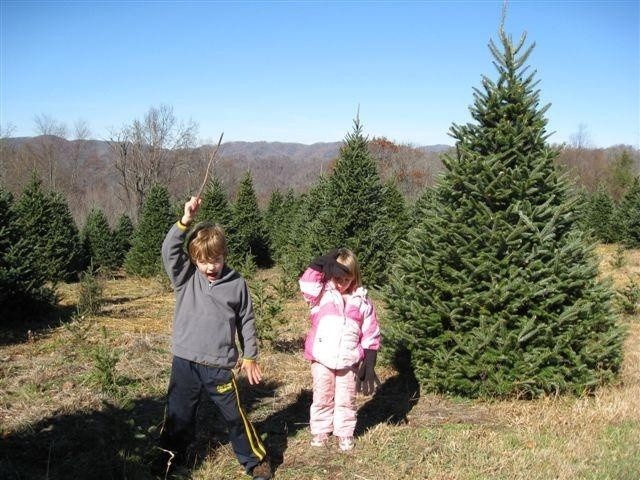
[297,246,382,452]
[150,195,277,480]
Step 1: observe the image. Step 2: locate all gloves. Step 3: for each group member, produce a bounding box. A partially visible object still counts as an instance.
[356,349,383,396]
[308,248,350,280]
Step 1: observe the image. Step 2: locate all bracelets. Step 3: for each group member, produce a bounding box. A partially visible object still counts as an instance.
[242,357,256,362]
[179,218,190,227]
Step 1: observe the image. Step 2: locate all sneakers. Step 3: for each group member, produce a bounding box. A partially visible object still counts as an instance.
[310,433,330,447]
[336,436,355,452]
[252,460,273,480]
